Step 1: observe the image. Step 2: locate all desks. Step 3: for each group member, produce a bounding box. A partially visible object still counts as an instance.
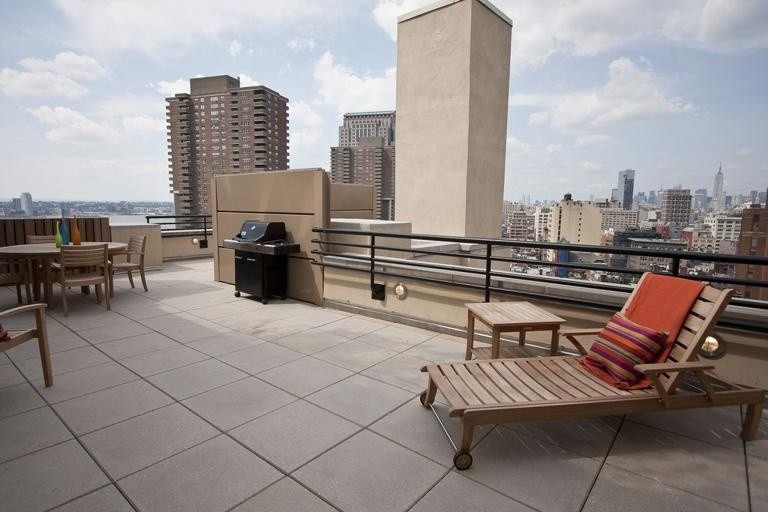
[464,301,567,359]
[0,243,129,309]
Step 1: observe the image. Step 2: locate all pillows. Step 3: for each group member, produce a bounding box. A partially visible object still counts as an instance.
[585,311,671,389]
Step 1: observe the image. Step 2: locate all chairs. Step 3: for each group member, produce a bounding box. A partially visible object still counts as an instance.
[26,233,56,300]
[0,302,53,387]
[0,254,33,305]
[419,271,768,470]
[111,234,149,292]
[49,244,111,317]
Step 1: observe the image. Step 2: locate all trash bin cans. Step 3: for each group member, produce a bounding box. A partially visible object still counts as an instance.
[232,220,289,304]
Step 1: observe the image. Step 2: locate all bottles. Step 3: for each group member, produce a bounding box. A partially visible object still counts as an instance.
[54,209,81,248]
